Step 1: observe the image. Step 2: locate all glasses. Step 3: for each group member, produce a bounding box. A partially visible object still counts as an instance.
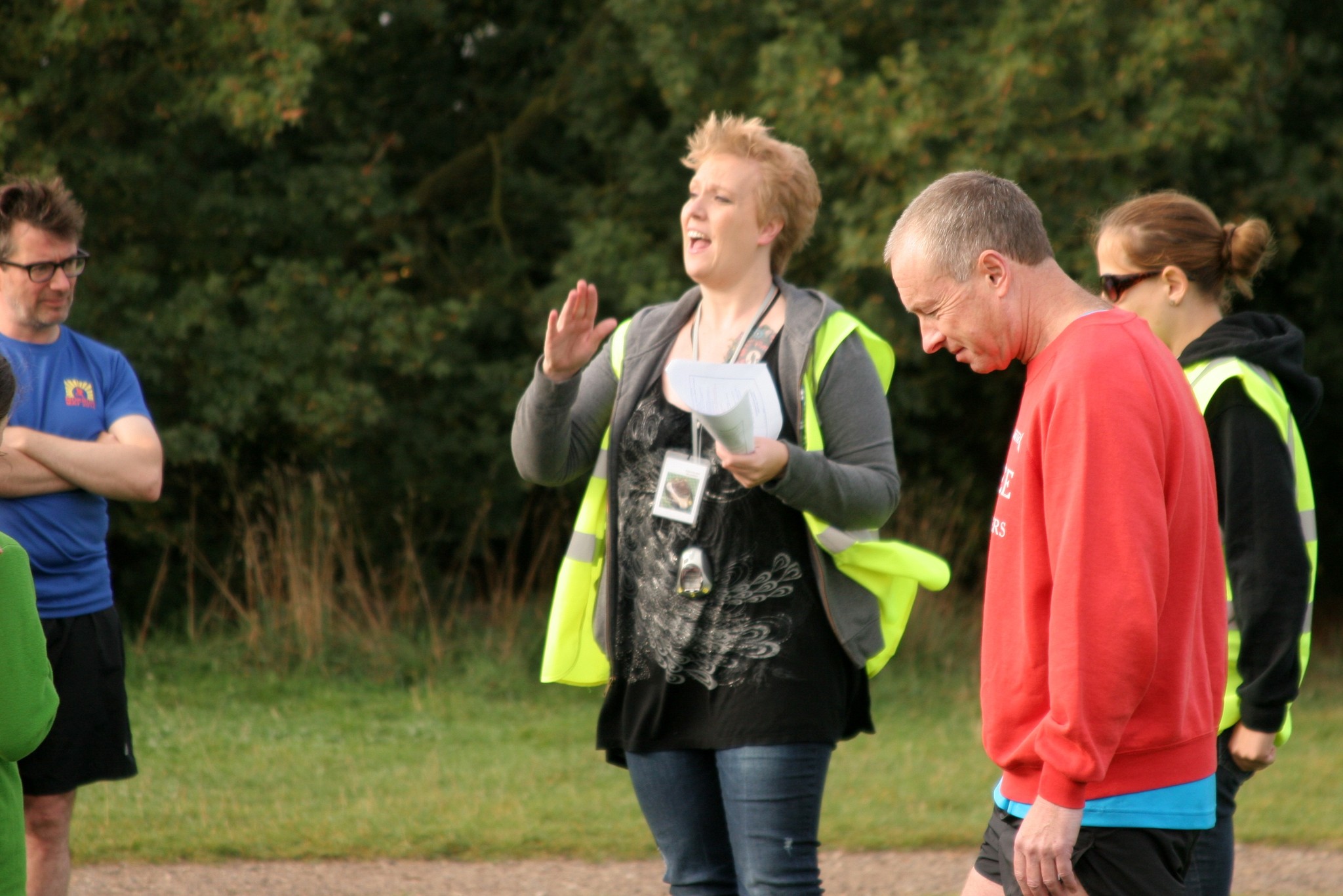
[0,250,89,282]
[1099,270,1194,302]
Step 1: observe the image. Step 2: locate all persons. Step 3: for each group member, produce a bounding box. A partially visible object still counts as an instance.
[512,112,949,896]
[0,174,161,896]
[882,168,1229,896]
[0,355,58,896]
[1091,194,1318,896]
